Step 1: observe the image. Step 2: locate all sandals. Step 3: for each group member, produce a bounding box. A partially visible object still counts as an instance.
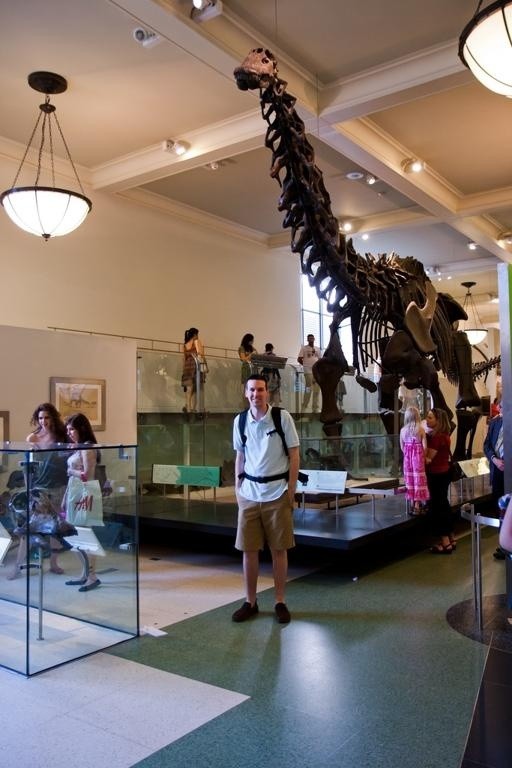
[430,540,456,554]
[409,507,428,515]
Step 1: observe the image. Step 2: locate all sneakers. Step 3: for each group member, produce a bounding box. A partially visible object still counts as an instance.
[79,579,100,591]
[65,579,87,585]
[232,598,258,622]
[49,568,64,574]
[275,603,290,623]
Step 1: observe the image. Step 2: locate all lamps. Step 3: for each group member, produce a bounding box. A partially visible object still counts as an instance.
[161,138,186,156]
[189,0,222,22]
[454,0,512,107]
[497,228,512,248]
[130,25,164,49]
[0,67,94,249]
[398,156,423,173]
[456,280,489,345]
[425,264,441,275]
[363,171,381,185]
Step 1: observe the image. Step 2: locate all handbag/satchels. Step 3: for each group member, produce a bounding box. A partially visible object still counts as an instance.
[448,461,464,482]
[80,462,113,491]
[198,357,208,373]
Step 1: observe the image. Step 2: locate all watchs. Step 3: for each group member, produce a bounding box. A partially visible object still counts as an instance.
[491,455,496,460]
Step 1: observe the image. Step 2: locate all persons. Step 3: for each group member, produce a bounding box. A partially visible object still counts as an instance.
[399,406,431,515]
[490,398,501,418]
[498,491,512,626]
[180,327,209,413]
[297,334,322,413]
[230,373,302,625]
[22,403,73,575]
[262,343,282,408]
[1,470,31,517]
[424,407,458,554]
[238,333,258,385]
[484,401,504,519]
[63,412,104,594]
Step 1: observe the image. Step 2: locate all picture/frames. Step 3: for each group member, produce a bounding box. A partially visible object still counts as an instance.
[49,376,108,437]
[0,409,12,474]
